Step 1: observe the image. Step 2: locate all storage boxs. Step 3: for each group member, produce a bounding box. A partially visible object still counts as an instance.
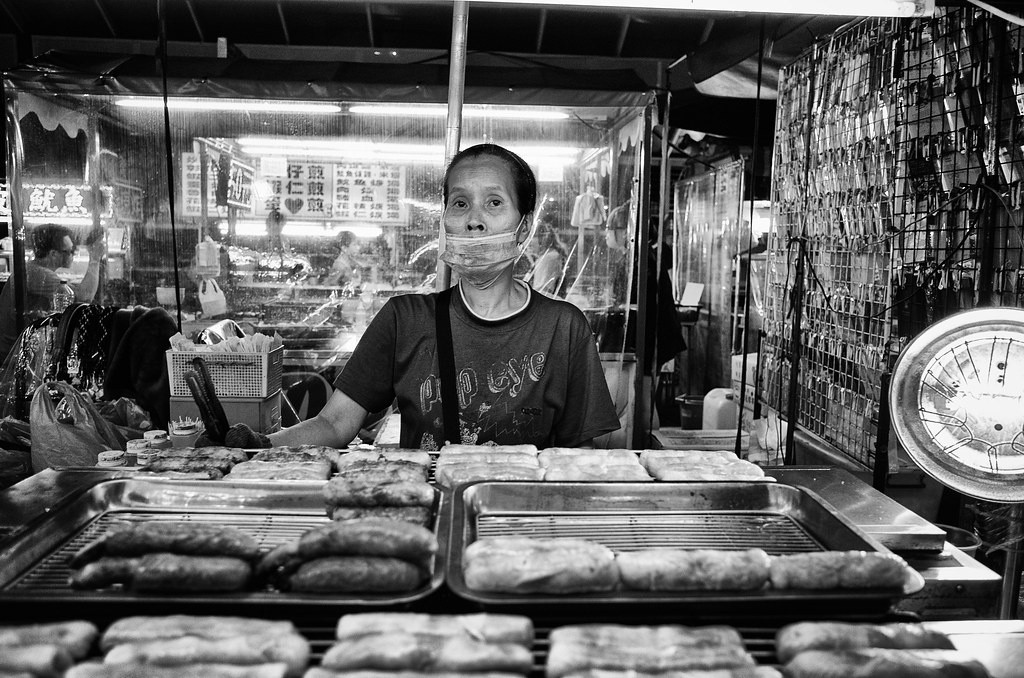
[168,342,286,435]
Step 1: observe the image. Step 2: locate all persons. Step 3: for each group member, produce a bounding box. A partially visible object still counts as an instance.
[254,210,291,253]
[526,221,567,299]
[320,230,364,285]
[194,143,622,450]
[599,206,687,429]
[0,223,105,367]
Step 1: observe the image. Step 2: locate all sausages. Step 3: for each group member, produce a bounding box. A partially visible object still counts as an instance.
[0,614,993,678]
[65,444,911,588]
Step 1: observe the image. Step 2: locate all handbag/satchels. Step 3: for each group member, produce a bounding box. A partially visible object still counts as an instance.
[30,381,126,474]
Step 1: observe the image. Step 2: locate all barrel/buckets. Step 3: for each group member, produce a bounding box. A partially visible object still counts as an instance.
[675,395,704,431]
[703,388,737,430]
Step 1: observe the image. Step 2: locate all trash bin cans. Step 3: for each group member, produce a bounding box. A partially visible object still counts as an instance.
[675,394,705,431]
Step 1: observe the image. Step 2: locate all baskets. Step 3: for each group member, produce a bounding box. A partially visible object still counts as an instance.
[164,346,284,398]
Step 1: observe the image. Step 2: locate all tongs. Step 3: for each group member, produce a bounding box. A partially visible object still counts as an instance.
[179,359,233,441]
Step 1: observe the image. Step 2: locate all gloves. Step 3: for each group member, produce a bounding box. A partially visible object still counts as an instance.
[195,423,272,448]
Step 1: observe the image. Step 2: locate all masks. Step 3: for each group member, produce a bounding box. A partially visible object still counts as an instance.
[439,215,524,292]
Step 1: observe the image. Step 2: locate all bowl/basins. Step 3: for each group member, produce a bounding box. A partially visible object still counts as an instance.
[157,286,185,306]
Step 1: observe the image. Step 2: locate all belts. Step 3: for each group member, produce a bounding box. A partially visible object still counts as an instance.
[53,304,114,386]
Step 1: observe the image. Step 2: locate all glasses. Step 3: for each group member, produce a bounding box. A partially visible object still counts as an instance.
[53,246,75,255]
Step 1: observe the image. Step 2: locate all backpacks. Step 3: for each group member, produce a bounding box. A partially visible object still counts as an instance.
[279,372,335,429]
